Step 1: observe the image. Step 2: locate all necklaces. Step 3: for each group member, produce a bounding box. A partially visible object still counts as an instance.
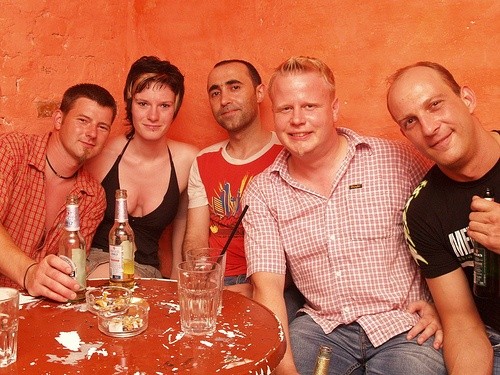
[46,154,77,179]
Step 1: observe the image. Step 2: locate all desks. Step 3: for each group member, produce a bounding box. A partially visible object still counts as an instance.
[0,278,287,375]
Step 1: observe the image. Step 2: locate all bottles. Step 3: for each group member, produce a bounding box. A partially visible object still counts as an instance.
[59,195,87,304]
[473,187,500,299]
[108,189,135,290]
[312,343,333,375]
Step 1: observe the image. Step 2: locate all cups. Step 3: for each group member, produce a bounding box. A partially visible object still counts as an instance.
[185,248,226,314]
[177,261,221,334]
[0,288,19,368]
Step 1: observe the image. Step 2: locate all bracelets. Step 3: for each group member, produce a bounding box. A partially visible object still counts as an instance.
[23,262,40,291]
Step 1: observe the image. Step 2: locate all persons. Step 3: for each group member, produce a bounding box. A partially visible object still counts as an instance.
[182,60,306,326]
[241,55,448,375]
[387,61,500,375]
[0,83,117,302]
[84,56,201,280]
